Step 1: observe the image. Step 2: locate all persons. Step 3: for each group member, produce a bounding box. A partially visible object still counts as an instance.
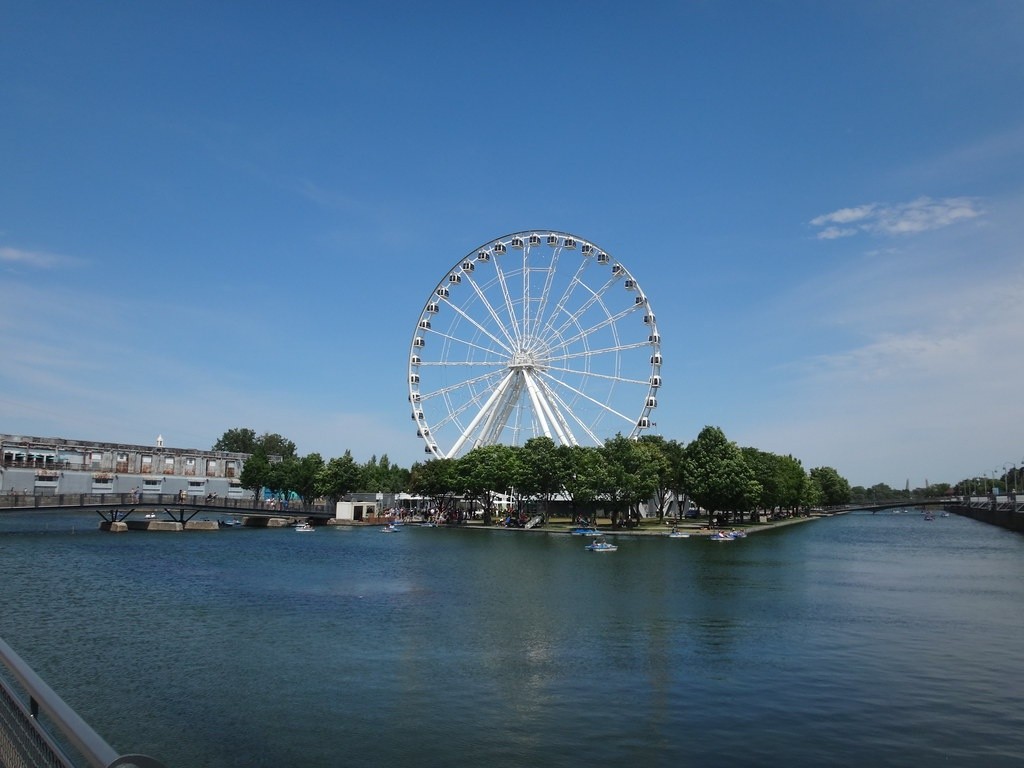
[709,520,714,529]
[602,538,607,546]
[131,486,140,504]
[11,487,15,495]
[389,507,399,515]
[249,495,255,501]
[272,498,276,508]
[23,488,27,506]
[593,538,598,546]
[719,530,730,538]
[618,518,624,527]
[541,513,544,524]
[178,490,183,500]
[207,492,218,503]
[717,513,722,522]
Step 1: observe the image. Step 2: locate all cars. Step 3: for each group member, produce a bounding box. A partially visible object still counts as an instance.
[685,510,701,519]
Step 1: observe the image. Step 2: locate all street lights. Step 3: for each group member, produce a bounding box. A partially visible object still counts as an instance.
[983,462,1016,496]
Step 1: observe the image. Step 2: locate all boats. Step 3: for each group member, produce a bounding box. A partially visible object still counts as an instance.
[384,520,405,532]
[711,531,747,540]
[585,544,618,552]
[920,509,935,520]
[941,512,950,517]
[421,522,434,527]
[145,514,155,519]
[291,517,315,532]
[226,518,241,526]
[668,533,690,538]
[571,529,603,537]
